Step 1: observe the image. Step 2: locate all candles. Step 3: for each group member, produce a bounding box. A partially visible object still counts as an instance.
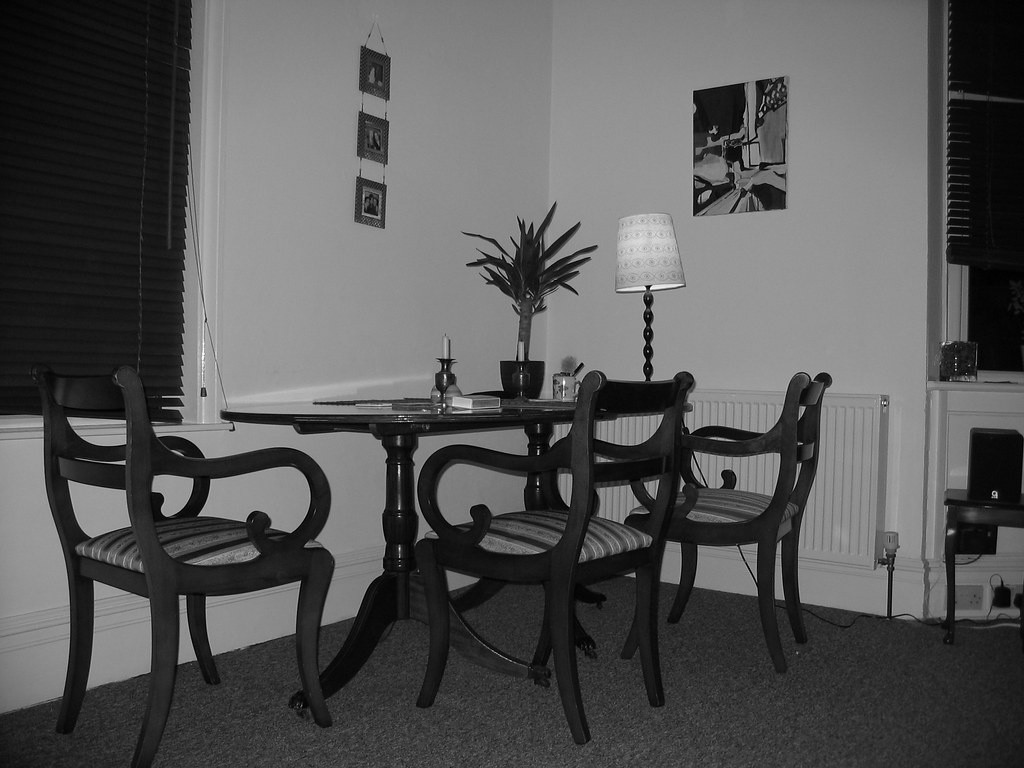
[442,333,450,359]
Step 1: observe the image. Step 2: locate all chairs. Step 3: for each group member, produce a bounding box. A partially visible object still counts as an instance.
[415,371,698,746]
[622,370,834,674]
[32,363,336,768]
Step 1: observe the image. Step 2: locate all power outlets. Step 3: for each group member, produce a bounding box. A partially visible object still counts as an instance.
[989,584,1024,611]
[943,584,983,611]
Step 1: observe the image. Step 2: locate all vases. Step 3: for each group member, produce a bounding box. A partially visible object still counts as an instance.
[435,357,456,402]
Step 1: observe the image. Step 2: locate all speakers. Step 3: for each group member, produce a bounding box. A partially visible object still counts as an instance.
[966,428,1024,505]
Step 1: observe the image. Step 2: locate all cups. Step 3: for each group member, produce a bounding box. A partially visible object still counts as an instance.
[553,377,583,402]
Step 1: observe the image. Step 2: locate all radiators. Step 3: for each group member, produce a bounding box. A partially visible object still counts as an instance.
[555,389,899,620]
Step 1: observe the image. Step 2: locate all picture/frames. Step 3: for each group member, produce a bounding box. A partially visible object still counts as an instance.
[359,45,391,101]
[356,111,390,166]
[353,176,387,229]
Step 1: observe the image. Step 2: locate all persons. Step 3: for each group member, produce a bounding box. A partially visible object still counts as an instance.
[372,131,381,150]
[368,66,383,85]
[364,194,379,216]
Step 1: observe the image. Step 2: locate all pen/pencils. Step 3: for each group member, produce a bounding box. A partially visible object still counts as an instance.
[572,363,584,377]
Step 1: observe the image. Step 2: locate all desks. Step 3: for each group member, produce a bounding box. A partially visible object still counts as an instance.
[220,397,694,721]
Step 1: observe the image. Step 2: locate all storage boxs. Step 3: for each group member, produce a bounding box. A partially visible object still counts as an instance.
[452,395,501,410]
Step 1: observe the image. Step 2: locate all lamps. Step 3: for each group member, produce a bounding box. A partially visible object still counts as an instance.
[615,212,686,381]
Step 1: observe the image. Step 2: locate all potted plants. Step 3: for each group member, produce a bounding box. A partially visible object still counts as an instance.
[460,200,599,400]
[940,341,979,382]
